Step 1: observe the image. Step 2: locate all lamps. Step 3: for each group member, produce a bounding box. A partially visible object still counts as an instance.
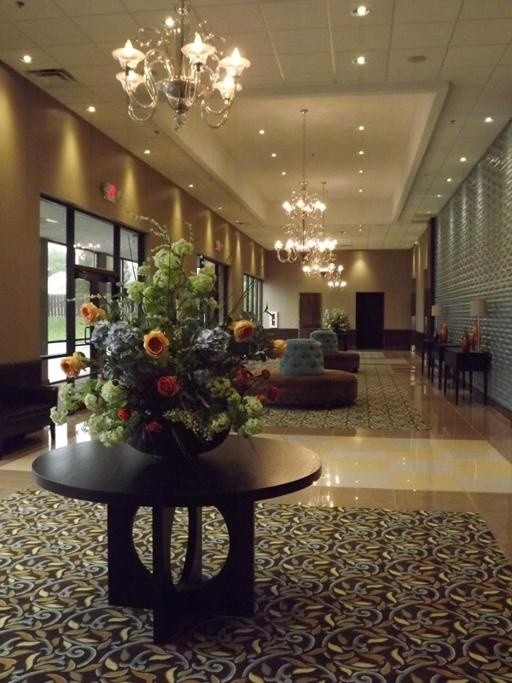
[273,109,348,290]
[431,305,445,336]
[111,1,253,129]
[471,303,488,353]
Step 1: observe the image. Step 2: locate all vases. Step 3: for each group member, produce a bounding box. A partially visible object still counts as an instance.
[121,416,233,457]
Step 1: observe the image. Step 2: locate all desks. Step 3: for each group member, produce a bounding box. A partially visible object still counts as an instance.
[412,337,492,406]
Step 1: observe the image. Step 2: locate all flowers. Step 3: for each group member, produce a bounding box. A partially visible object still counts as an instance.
[49,214,287,482]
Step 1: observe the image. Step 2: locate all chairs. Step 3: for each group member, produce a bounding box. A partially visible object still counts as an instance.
[251,339,359,409]
[0,360,59,450]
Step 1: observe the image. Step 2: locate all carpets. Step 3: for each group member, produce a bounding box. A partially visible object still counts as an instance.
[0,484,511,682]
[244,349,428,430]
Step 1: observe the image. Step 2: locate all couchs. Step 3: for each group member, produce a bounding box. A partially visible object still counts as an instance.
[310,330,359,372]
